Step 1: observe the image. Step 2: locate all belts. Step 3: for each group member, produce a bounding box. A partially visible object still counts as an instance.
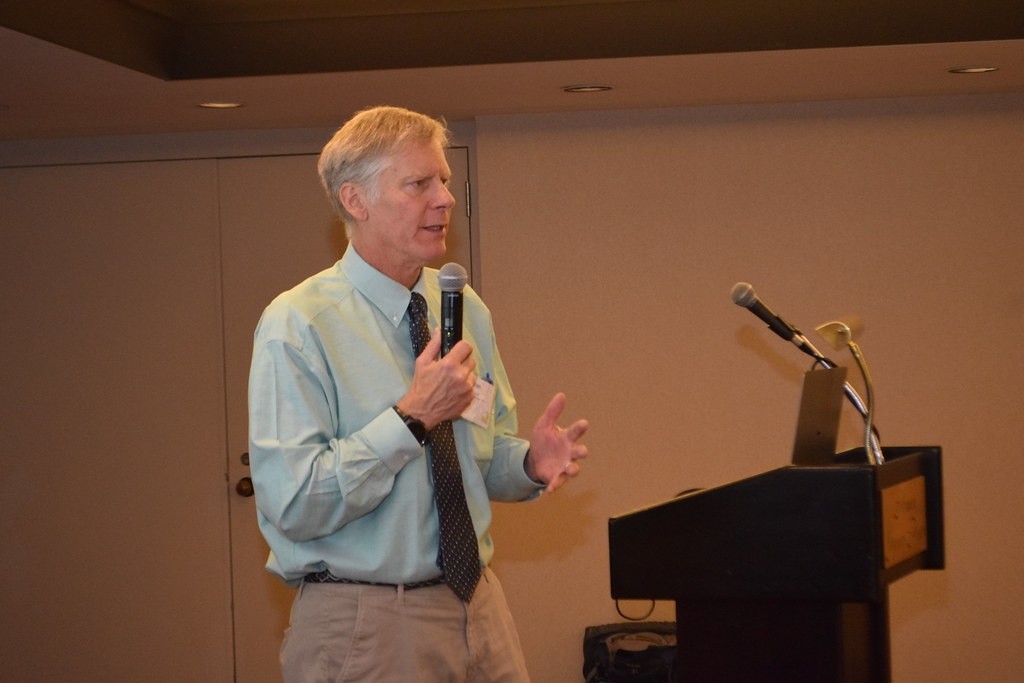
[303,572,447,591]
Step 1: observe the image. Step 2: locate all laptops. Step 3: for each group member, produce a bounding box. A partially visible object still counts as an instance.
[791,366,848,467]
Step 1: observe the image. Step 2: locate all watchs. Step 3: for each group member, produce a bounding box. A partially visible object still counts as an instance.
[393,405,431,447]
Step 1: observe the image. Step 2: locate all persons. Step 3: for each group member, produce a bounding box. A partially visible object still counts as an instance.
[248,107,589,683]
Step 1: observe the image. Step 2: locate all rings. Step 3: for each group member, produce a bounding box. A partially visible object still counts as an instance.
[565,461,571,472]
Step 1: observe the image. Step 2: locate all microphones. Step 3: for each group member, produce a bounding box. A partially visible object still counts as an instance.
[729,282,816,359]
[437,262,468,359]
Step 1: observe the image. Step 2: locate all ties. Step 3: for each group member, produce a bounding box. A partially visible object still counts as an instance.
[407,292,482,603]
